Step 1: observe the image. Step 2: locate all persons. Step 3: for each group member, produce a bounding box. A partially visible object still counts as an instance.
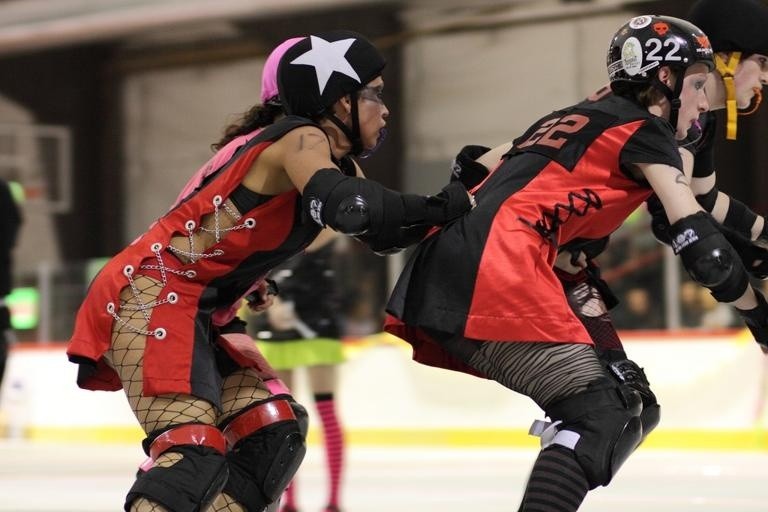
[688,0,768,237]
[0,176,28,382]
[605,237,668,327]
[386,1,768,512]
[240,229,381,512]
[67,36,517,511]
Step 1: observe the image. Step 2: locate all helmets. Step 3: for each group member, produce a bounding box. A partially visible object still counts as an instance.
[261,31,386,118]
[606,0,768,96]
